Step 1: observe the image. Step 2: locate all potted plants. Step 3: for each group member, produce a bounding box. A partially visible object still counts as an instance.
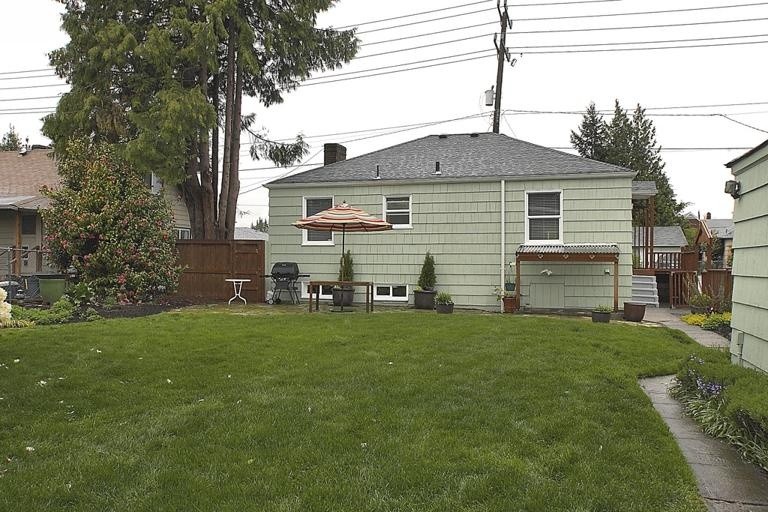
[434,288,454,314]
[689,294,712,315]
[591,304,614,323]
[413,249,437,309]
[492,288,516,313]
[332,250,354,306]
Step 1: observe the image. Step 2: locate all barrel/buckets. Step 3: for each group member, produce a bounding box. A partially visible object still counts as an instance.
[413,289,438,309]
[333,288,354,306]
[503,297,517,313]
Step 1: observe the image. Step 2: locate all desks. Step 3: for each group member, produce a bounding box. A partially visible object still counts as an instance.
[225,278,251,305]
[309,280,373,313]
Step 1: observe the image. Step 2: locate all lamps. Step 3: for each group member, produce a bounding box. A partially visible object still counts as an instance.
[724,179,740,200]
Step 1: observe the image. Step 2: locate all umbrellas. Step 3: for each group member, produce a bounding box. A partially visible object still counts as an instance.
[291,200,394,310]
